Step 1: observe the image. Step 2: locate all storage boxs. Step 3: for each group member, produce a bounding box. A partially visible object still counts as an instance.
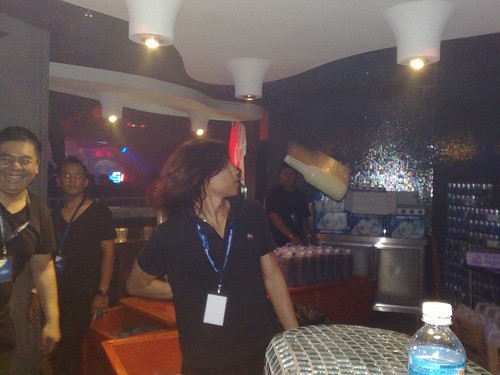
[313,186,425,240]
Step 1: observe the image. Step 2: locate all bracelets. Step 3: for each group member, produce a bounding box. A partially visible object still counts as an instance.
[98,292,108,296]
[31,288,40,294]
[99,287,109,292]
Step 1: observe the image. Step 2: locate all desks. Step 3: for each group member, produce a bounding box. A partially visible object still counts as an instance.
[263,326,493,375]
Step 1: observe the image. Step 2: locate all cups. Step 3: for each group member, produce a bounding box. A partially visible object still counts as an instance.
[116,228,128,240]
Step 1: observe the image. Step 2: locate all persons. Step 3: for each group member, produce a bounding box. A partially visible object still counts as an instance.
[124,137,299,375]
[266,165,316,249]
[0,126,60,375]
[27,157,118,375]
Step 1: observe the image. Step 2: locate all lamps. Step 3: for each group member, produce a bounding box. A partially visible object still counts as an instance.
[100,94,124,122]
[226,59,273,101]
[383,1,459,70]
[124,0,186,48]
[190,111,210,134]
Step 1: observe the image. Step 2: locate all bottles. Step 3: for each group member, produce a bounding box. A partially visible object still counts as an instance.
[282,155,349,203]
[408,300,467,375]
[274,244,354,287]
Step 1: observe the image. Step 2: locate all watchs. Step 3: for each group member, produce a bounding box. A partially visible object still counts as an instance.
[289,233,295,239]
